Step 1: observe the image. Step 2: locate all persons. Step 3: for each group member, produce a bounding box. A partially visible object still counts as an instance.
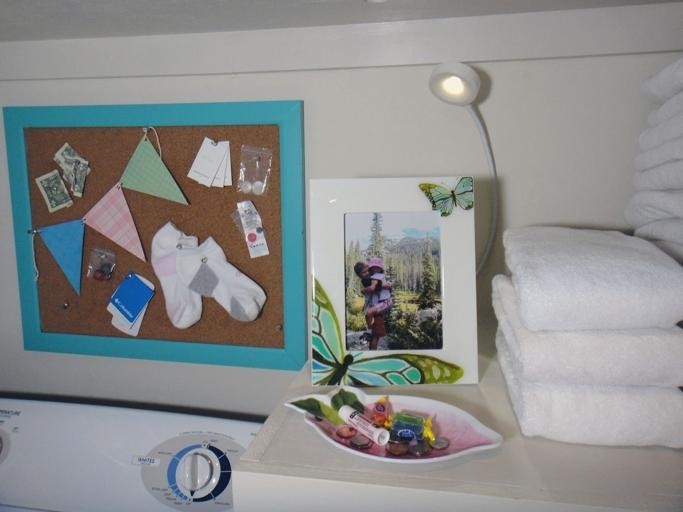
[354,262,391,351]
[360,258,390,341]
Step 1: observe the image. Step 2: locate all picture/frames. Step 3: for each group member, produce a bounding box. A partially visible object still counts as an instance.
[306,175,479,390]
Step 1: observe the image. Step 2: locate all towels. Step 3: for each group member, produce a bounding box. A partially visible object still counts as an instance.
[490,273,682,390]
[623,57,683,266]
[498,225,683,332]
[494,331,682,452]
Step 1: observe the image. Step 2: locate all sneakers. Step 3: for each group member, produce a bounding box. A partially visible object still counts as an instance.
[359,332,371,340]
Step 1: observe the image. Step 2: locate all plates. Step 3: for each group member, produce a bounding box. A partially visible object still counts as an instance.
[284,386,503,463]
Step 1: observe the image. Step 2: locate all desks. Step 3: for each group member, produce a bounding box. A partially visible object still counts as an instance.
[229,360,681,509]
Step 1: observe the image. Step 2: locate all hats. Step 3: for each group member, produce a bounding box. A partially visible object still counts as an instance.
[369,258,385,272]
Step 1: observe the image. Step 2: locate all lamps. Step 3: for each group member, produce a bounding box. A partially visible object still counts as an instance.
[427,60,499,277]
